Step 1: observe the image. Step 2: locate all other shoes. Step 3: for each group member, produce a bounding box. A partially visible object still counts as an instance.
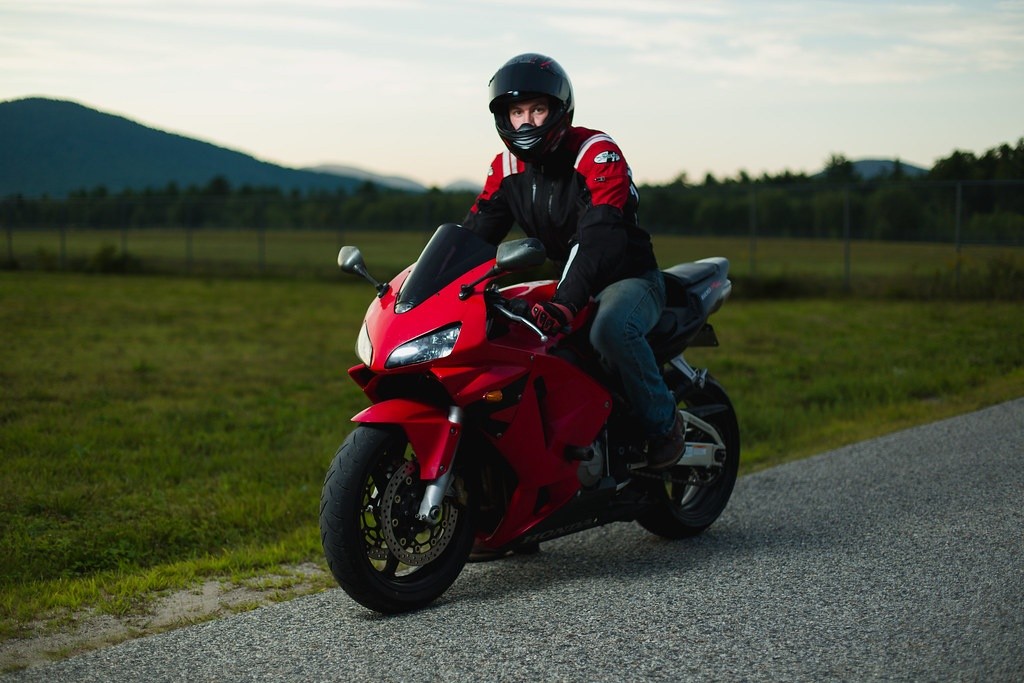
[646,412,686,470]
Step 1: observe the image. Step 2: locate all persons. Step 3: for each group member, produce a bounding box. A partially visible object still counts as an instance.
[463,53,688,563]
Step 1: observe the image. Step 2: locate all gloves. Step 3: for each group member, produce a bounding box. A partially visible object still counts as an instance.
[530,299,581,339]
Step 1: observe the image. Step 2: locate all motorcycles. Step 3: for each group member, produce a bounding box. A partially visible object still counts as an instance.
[319,223,741,615]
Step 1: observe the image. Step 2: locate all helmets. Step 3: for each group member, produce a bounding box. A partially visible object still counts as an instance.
[487,52,574,163]
